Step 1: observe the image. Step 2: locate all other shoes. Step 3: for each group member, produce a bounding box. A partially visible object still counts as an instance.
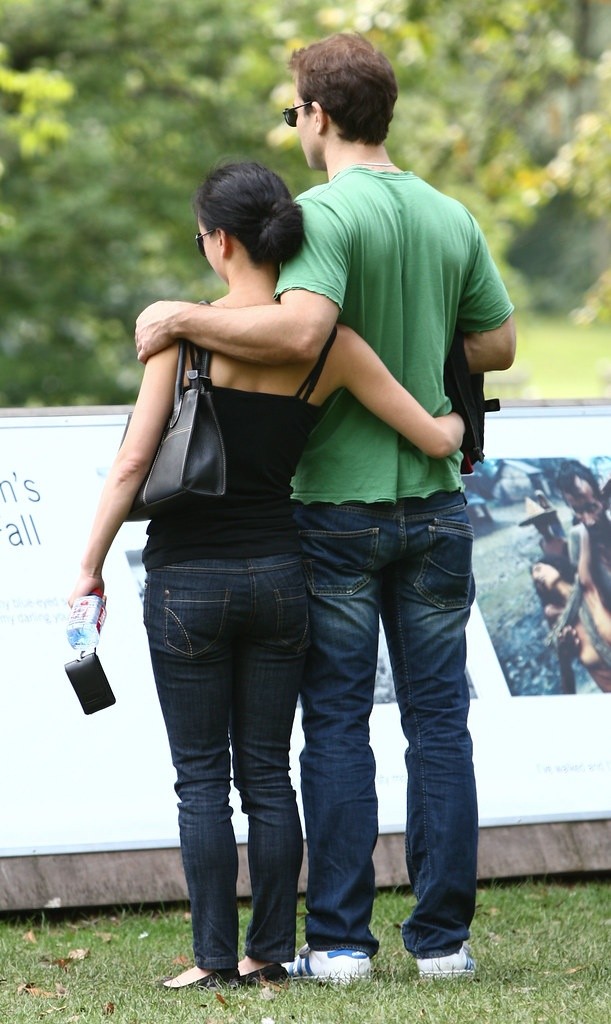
[239,963,292,986]
[162,969,239,991]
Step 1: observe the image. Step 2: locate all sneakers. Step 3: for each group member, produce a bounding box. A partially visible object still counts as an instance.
[416,939,474,981]
[283,942,371,985]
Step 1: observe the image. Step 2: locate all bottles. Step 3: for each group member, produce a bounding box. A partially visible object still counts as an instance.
[66,589,107,650]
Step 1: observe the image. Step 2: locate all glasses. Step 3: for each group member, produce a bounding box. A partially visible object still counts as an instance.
[195,224,239,258]
[282,99,322,128]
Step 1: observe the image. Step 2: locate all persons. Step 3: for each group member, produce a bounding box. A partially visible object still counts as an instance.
[519,459,611,693]
[134,33,514,990]
[68,164,466,994]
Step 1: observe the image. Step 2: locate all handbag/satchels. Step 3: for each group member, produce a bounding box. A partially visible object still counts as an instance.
[117,301,226,521]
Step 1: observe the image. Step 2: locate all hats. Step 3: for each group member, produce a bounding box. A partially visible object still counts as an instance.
[519,496,558,526]
